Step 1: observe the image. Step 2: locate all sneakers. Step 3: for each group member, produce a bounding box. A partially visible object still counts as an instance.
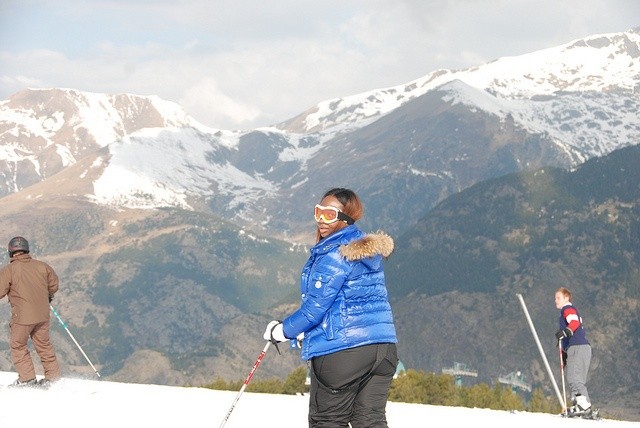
[37,378,59,388]
[564,403,592,415]
[8,378,37,388]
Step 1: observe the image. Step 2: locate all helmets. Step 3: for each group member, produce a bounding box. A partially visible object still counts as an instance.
[8,237,29,257]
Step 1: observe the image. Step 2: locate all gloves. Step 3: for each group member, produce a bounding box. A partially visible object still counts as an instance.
[555,327,573,339]
[264,321,290,343]
[296,332,304,348]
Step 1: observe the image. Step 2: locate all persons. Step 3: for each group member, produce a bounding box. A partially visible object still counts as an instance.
[263,187,398,427]
[0,236,62,390]
[554,287,593,415]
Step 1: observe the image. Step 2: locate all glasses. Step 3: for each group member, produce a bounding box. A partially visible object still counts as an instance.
[314,204,355,225]
